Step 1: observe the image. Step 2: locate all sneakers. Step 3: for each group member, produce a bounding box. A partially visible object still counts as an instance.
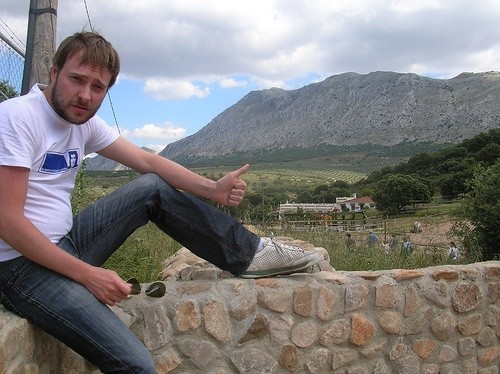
[240,236,323,279]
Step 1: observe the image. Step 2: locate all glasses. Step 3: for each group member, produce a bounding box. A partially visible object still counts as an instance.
[123,276,166,298]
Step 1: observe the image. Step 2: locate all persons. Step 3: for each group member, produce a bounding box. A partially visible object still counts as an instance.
[390,234,399,250]
[0,31,325,374]
[366,230,379,250]
[401,237,413,256]
[381,240,389,256]
[447,241,461,259]
[345,233,356,249]
[415,220,422,234]
